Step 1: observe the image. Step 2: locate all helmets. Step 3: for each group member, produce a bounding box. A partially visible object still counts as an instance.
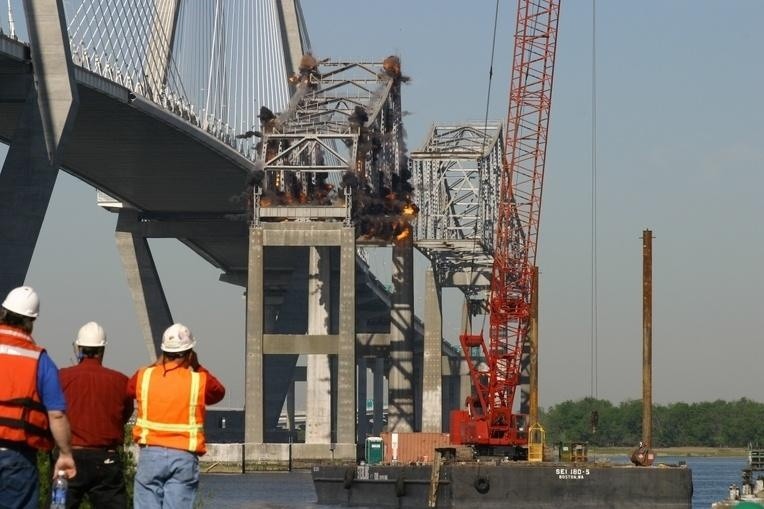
[160,323,197,353]
[76,321,107,347]
[1,286,39,318]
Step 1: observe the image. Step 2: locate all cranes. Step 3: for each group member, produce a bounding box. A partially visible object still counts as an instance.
[447,0,566,458]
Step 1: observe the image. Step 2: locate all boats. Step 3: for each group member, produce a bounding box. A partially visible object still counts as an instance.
[306,456,693,509]
[711,443,764,509]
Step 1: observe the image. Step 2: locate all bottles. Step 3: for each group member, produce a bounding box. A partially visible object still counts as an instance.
[48,469,69,509]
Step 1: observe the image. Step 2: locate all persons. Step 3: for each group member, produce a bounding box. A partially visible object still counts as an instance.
[259,171,419,242]
[300,50,317,75]
[384,55,400,79]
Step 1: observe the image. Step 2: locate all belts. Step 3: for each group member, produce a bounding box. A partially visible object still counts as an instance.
[72,445,102,451]
